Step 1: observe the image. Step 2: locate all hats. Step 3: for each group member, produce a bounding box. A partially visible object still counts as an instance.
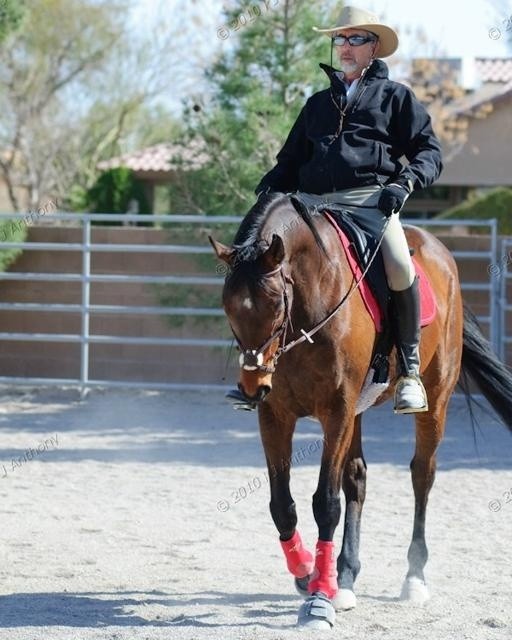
[311,6,399,58]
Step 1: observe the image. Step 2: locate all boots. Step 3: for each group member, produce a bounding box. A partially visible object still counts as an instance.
[386,273,426,414]
[225,381,256,411]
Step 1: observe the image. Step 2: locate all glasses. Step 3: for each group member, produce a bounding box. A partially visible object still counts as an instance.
[332,33,376,46]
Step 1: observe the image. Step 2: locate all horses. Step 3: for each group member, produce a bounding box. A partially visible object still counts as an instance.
[208,191,512,630]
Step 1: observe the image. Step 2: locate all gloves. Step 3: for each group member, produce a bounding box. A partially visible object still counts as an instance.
[377,175,414,218]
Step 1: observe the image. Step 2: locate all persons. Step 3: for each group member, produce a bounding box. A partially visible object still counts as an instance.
[225,5,445,414]
